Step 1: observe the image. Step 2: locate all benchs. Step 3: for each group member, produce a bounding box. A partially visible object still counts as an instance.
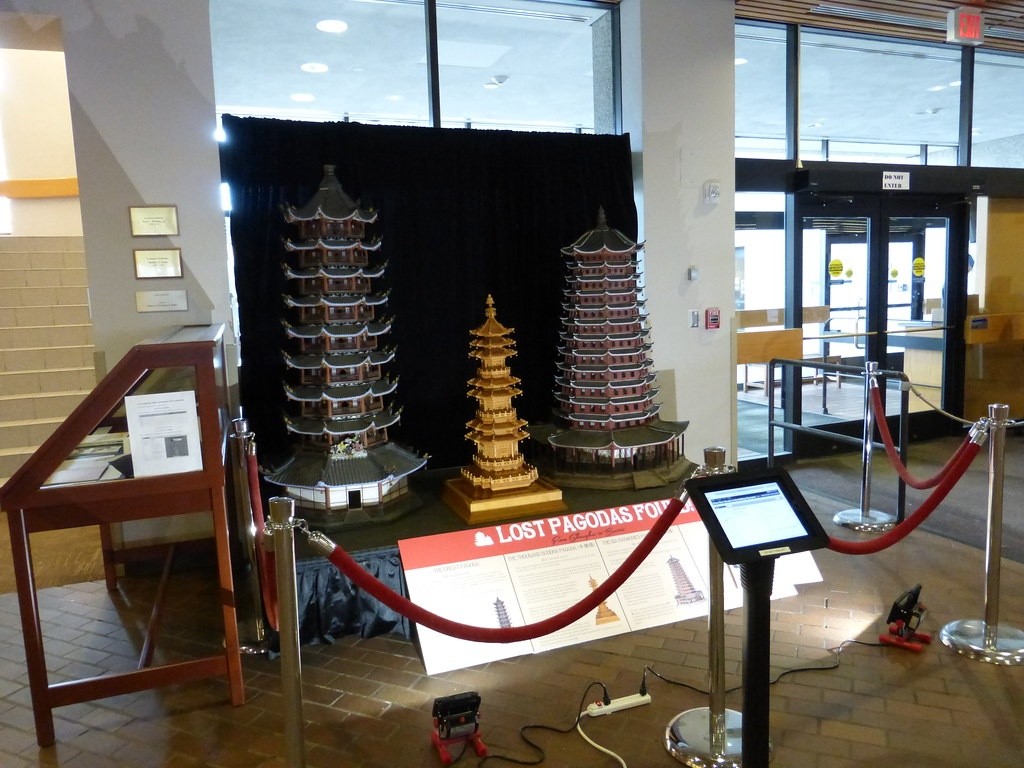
[743,352,842,397]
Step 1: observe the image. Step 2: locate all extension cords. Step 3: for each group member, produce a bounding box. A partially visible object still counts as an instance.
[587,693,652,716]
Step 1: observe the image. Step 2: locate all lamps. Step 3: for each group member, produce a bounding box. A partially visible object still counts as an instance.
[886,584,928,633]
[432,691,481,740]
